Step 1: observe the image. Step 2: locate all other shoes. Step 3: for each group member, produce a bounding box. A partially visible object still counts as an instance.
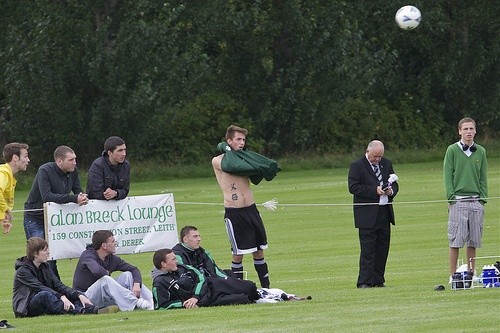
[94,304,120,314]
[358,284,372,288]
[138,299,153,311]
[374,283,386,287]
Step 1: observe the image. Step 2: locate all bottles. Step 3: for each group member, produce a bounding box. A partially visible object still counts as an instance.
[452,272,463,289]
[482,269,497,287]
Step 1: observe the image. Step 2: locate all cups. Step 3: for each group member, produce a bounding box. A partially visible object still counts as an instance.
[464,271,473,288]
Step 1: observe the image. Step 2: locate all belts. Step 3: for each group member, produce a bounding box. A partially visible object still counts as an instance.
[458,196,479,202]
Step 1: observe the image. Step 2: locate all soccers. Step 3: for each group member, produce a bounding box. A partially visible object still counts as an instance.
[395,5,422,31]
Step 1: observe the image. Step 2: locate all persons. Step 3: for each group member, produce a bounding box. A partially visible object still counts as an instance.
[24,146,88,282]
[13,237,98,318]
[73,230,152,311]
[86,137,131,201]
[151,226,260,310]
[0,143,30,329]
[212,125,278,295]
[443,118,488,283]
[348,140,399,288]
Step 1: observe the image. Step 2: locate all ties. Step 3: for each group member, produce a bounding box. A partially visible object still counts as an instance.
[373,165,383,185]
